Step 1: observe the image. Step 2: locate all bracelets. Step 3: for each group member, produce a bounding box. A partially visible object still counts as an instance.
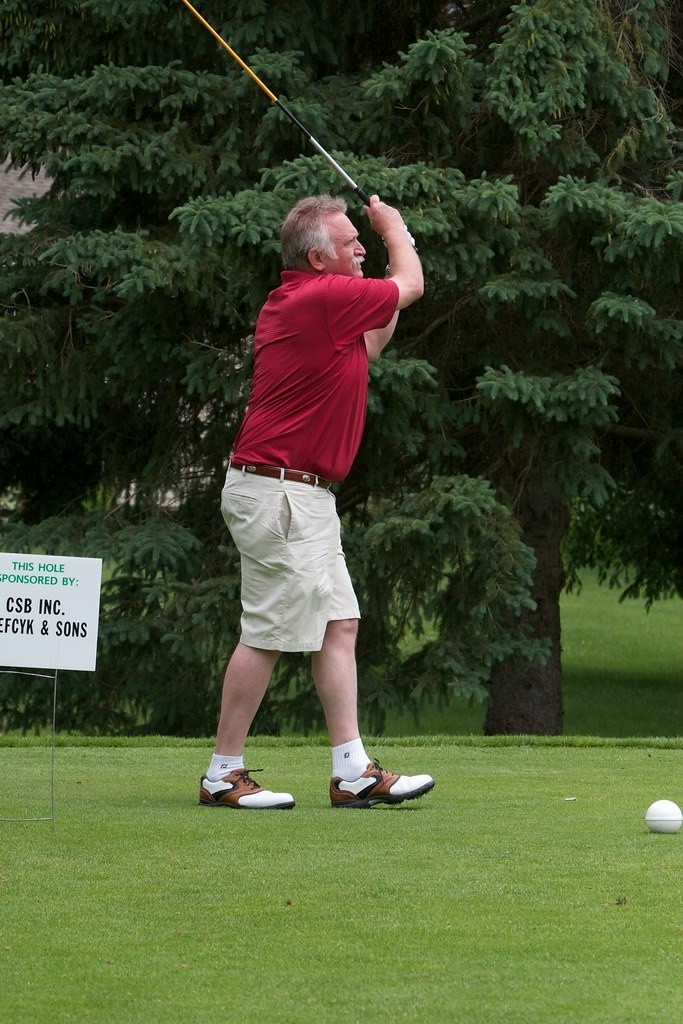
[381,224,416,248]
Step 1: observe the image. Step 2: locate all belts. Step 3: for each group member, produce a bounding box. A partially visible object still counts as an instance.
[230,460,332,490]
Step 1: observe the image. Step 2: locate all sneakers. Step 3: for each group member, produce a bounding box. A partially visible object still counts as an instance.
[198,766,295,808]
[329,758,435,808]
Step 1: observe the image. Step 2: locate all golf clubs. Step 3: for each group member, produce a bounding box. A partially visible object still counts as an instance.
[183,1,420,253]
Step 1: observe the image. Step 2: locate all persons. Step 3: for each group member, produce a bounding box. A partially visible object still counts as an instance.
[197,194,436,809]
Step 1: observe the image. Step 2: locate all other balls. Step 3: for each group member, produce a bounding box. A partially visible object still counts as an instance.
[644,799,682,832]
